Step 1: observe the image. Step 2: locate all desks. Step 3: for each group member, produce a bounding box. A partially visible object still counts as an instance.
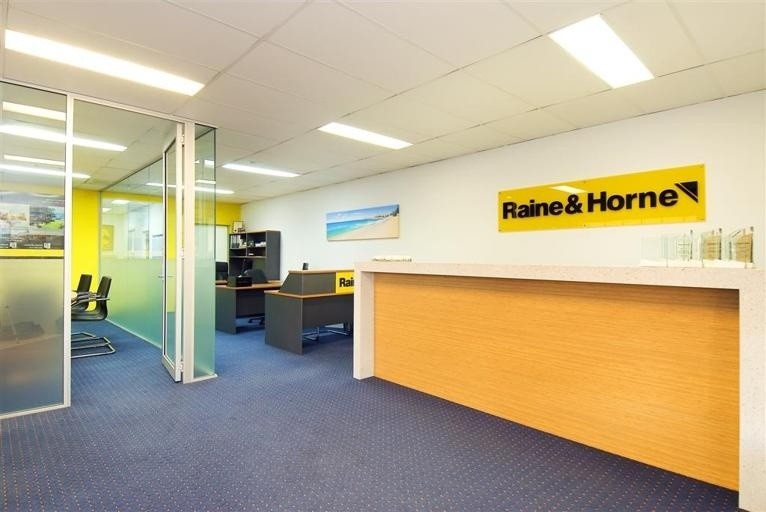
[215,271,353,356]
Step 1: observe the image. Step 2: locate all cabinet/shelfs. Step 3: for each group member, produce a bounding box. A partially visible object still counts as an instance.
[229,230,280,279]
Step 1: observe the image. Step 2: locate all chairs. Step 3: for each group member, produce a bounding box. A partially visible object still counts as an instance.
[216,262,228,280]
[72,275,116,359]
